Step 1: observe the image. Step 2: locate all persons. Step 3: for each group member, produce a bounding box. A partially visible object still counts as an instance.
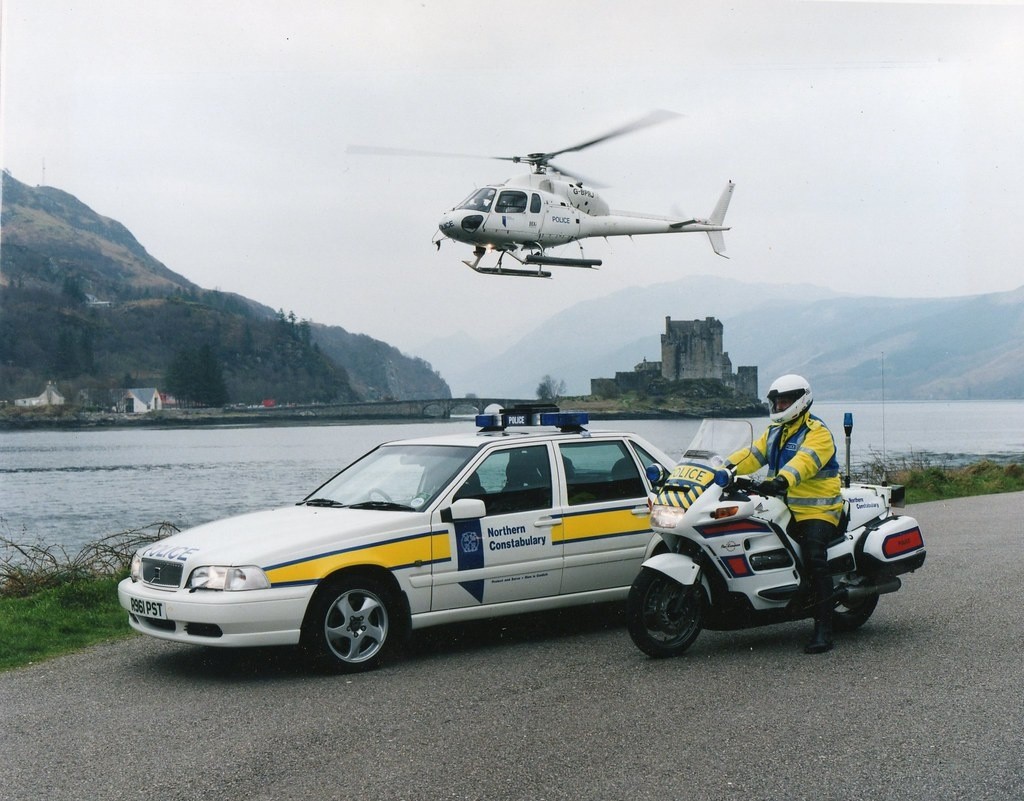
[712,374,843,653]
[474,197,484,209]
[487,191,502,211]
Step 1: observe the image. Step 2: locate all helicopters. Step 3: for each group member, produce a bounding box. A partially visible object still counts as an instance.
[343,113,740,276]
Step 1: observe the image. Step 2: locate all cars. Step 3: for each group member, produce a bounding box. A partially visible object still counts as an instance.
[115,405,685,673]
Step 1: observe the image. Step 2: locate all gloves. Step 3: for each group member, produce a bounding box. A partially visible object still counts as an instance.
[757,475,788,498]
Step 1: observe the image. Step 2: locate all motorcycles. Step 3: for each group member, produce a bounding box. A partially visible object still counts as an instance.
[626,411,926,657]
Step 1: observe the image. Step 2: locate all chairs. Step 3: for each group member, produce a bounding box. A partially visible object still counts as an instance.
[424,463,486,499]
[500,453,646,484]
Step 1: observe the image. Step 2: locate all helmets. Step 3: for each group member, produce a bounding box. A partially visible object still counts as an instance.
[767,374,813,424]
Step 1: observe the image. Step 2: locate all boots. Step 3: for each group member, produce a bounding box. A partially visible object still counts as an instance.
[805,568,833,652]
